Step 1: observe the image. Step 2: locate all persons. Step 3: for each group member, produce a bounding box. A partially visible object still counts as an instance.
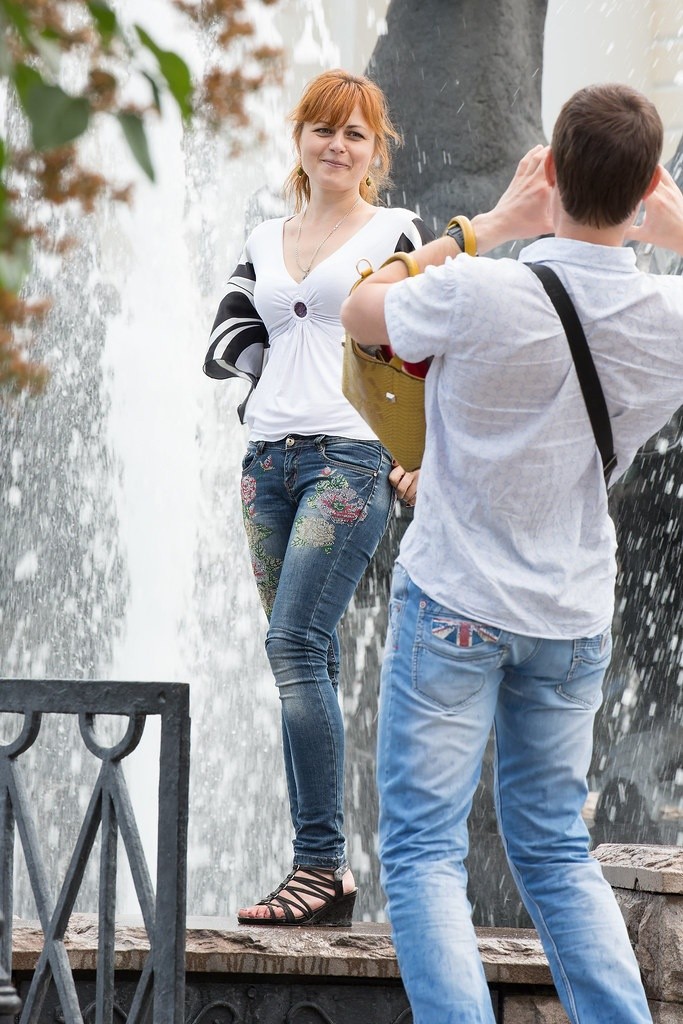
[340,81,683,1024]
[200,69,438,929]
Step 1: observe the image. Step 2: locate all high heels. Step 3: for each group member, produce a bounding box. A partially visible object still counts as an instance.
[238,860,359,927]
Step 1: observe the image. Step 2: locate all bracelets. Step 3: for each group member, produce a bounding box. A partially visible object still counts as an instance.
[447,224,479,256]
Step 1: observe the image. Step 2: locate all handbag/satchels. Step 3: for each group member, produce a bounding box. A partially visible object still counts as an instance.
[341,215,476,473]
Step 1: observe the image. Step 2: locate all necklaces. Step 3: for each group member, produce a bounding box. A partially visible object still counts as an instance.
[295,199,361,280]
